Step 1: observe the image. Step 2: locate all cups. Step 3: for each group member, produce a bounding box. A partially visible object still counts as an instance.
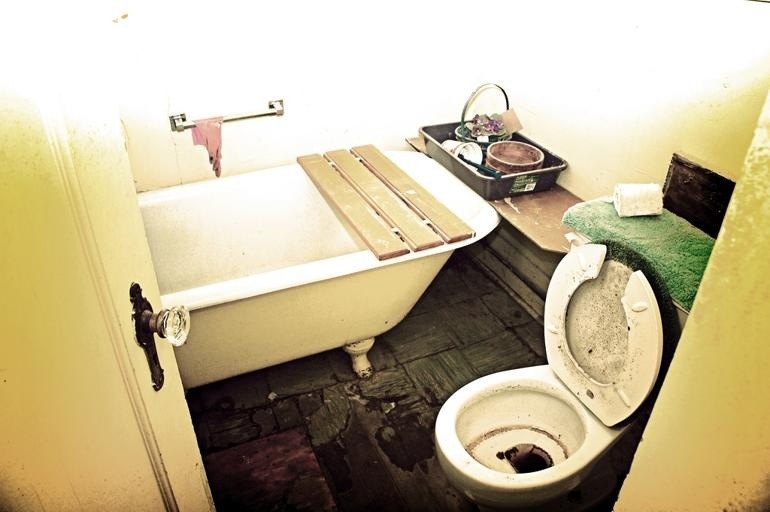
[441,139,483,171]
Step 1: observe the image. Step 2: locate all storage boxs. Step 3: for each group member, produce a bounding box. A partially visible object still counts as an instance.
[418,119,569,201]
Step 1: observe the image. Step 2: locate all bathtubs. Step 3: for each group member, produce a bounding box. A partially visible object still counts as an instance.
[136,153,500,391]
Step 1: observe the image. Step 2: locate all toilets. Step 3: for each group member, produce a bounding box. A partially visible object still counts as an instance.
[434,196,716,512]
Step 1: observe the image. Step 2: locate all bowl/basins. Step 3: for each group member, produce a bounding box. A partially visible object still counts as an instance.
[485,141,546,174]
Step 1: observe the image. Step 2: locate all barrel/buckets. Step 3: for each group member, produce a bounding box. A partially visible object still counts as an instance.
[454,83,511,152]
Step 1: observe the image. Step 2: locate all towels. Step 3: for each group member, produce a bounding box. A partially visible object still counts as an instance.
[562,196,717,313]
